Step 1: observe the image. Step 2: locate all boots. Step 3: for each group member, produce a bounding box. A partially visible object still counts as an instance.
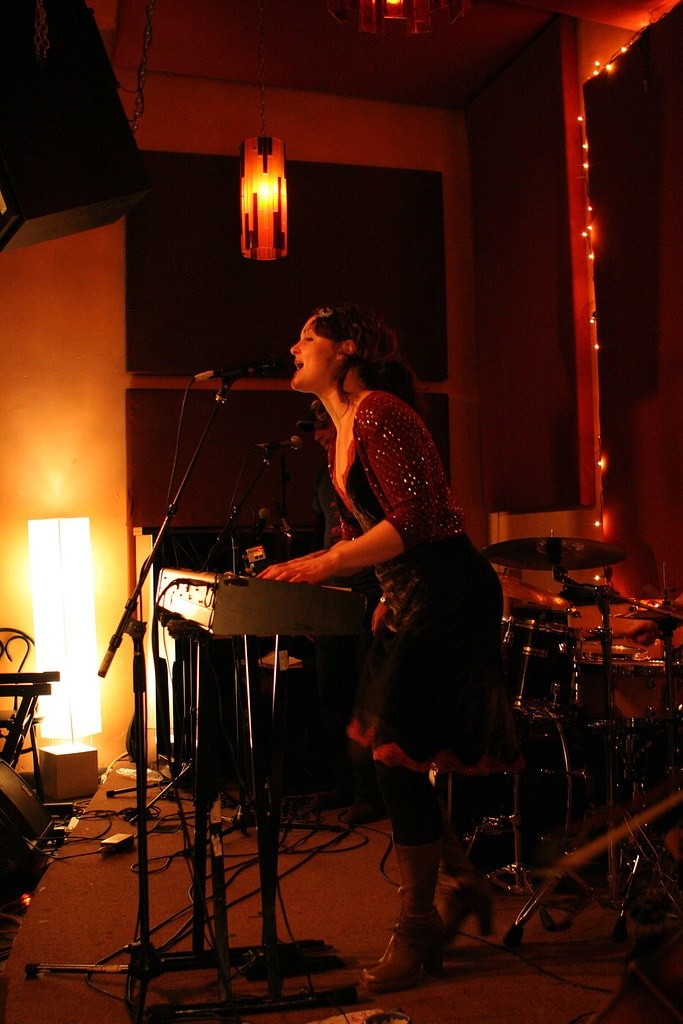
[434,829,493,946]
[360,836,446,992]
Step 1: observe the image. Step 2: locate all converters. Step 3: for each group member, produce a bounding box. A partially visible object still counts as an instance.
[101,834,135,855]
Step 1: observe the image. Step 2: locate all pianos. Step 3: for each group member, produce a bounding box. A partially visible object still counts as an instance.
[158,561,361,1016]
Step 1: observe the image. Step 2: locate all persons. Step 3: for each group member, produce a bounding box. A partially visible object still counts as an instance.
[296,397,389,827]
[251,302,505,992]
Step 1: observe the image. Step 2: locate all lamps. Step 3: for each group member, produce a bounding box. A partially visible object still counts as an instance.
[239,0,287,260]
[28,517,102,802]
[324,0,475,38]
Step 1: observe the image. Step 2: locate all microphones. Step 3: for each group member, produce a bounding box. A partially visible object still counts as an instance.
[255,435,303,451]
[193,360,276,381]
[254,507,270,535]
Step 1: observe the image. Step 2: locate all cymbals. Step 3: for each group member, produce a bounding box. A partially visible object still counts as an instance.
[608,604,683,619]
[501,577,571,607]
[479,536,627,570]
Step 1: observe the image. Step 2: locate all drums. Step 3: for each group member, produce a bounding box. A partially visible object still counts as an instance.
[434,698,589,894]
[500,613,582,722]
[576,641,683,727]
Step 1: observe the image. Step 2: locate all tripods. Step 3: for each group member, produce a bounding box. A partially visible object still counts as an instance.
[182,769,343,858]
[505,570,683,951]
[478,767,560,928]
[25,379,256,1024]
